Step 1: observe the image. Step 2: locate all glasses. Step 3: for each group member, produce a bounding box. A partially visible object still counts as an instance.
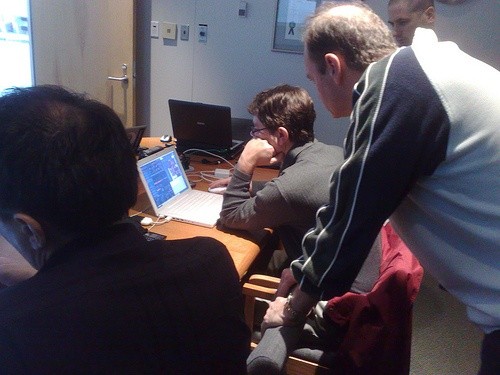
[249,126,268,137]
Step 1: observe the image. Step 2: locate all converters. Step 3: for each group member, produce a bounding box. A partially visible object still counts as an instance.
[215,169,230,178]
[142,146,163,156]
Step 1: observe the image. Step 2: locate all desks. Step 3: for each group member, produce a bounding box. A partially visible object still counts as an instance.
[129,137,284,280]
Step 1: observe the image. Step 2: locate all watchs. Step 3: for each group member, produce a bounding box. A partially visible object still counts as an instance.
[284,292,299,322]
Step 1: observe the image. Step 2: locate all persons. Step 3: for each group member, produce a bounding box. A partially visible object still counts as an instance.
[387,0,436,47]
[0,84,252,375]
[260,0,500,375]
[218,83,382,331]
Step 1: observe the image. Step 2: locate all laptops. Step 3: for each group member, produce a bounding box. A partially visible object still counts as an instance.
[168,99,245,156]
[136,145,223,229]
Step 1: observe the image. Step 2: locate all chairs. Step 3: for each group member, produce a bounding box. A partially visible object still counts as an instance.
[231,117,252,142]
[242,220,426,375]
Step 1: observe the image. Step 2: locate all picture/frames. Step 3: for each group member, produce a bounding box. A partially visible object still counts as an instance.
[270,0,321,55]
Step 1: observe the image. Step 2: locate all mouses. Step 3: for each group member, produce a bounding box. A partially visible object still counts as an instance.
[160,134,172,142]
[208,184,226,193]
